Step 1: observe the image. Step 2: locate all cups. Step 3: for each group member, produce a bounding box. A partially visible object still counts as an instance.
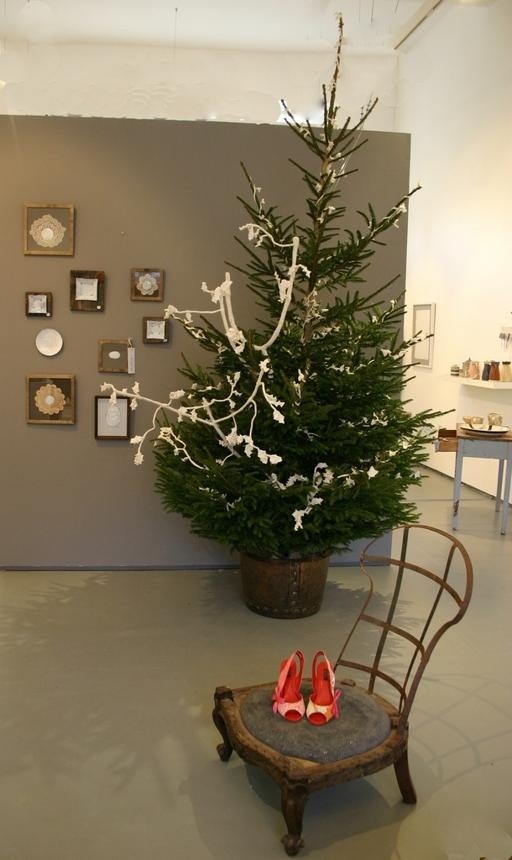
[488,412,503,426]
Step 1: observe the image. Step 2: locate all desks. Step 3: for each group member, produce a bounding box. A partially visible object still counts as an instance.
[434,420,512,538]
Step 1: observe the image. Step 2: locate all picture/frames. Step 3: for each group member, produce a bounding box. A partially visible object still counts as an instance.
[21,201,76,257]
[24,373,79,427]
[141,316,169,345]
[25,288,55,318]
[409,299,435,371]
[95,338,134,375]
[67,268,108,313]
[130,266,165,303]
[92,394,133,442]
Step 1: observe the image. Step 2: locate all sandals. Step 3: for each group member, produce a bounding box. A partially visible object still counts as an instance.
[305,650,341,725]
[273,648,306,721]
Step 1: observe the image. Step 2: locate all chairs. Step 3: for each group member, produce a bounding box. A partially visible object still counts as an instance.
[208,522,476,859]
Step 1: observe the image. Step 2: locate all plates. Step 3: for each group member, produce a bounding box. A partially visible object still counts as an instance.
[459,423,511,436]
[34,327,63,356]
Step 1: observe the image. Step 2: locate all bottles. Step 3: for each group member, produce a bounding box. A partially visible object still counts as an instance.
[449,356,512,382]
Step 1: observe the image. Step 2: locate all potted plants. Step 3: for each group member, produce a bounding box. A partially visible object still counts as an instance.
[92,9,463,620]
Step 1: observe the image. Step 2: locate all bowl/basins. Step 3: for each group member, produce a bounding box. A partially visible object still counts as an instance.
[463,416,484,424]
